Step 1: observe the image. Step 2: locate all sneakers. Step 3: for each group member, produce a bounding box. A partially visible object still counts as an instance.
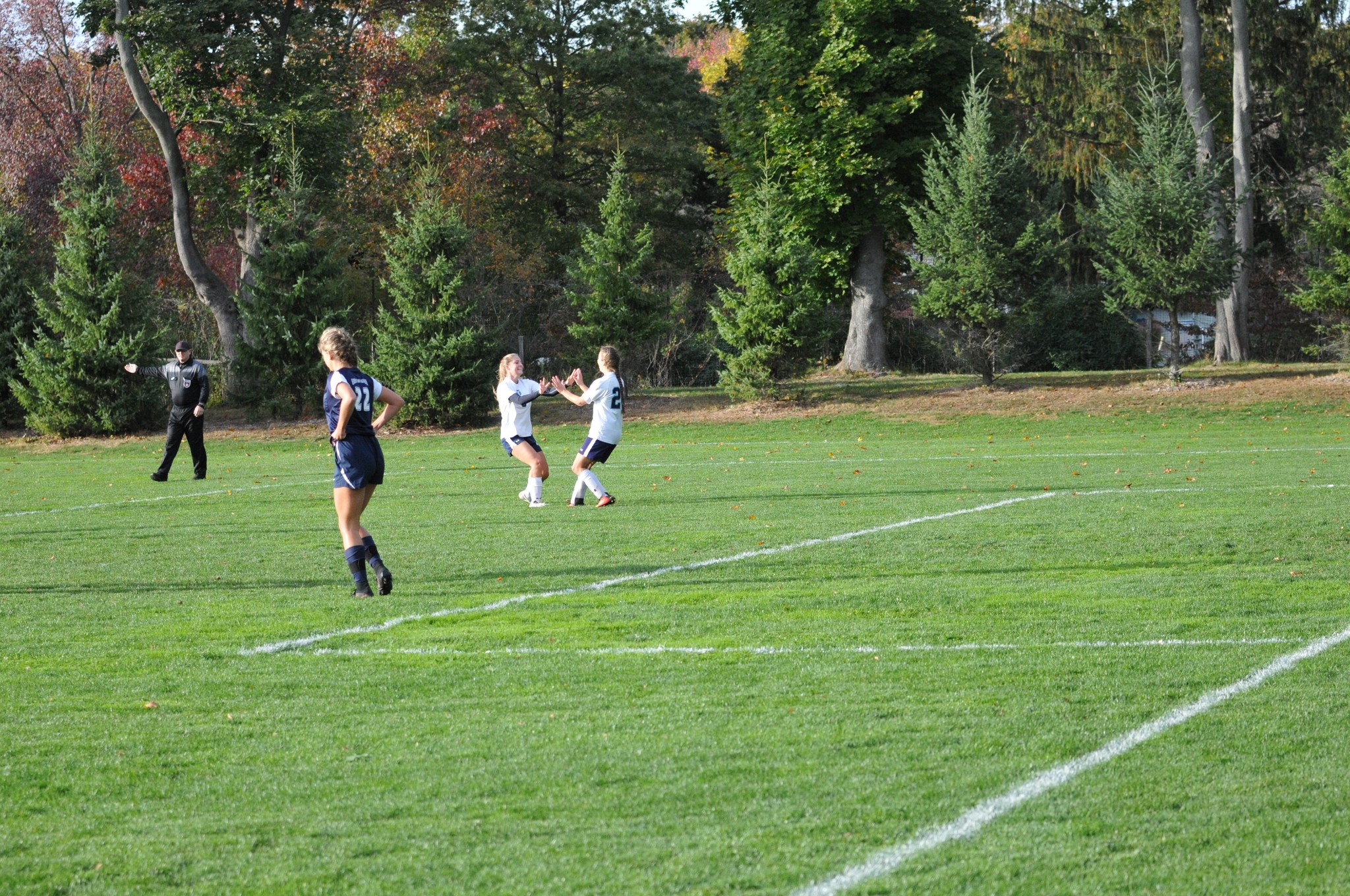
[348,588,374,598]
[567,503,574,507]
[529,502,549,507]
[596,495,616,507]
[519,490,531,503]
[376,570,393,596]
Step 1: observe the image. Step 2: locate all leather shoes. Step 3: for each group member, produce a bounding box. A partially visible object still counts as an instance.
[150,473,168,481]
[192,473,206,480]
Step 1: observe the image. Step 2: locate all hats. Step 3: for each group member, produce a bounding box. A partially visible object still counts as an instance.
[176,341,191,350]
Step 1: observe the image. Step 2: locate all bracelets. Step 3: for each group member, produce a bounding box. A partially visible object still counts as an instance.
[198,402,206,407]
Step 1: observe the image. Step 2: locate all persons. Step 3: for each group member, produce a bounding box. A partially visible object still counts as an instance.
[124,340,211,482]
[496,353,576,507]
[551,346,626,508]
[316,326,406,601]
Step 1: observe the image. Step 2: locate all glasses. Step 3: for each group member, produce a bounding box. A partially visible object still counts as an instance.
[177,349,187,352]
